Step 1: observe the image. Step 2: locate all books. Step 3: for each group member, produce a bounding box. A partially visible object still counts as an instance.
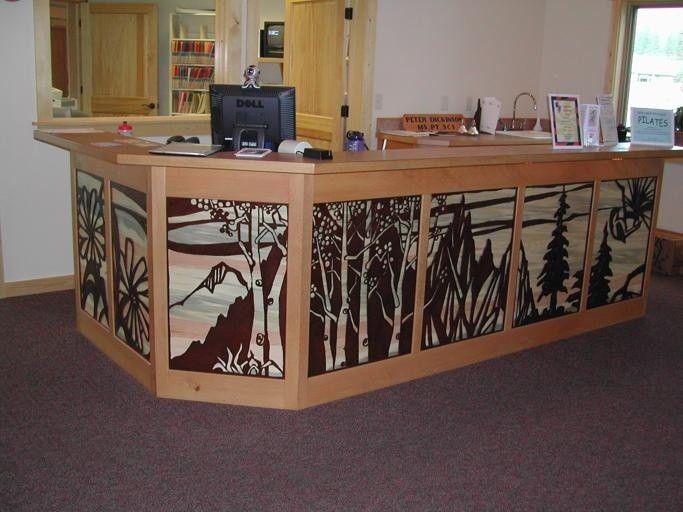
[170,68,213,90]
[172,39,216,67]
[171,92,210,114]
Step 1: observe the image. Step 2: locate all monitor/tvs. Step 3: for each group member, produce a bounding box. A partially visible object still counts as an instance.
[209,84,296,158]
[263,20,285,58]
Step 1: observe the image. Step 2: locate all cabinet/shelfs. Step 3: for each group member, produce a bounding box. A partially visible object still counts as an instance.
[165,8,217,112]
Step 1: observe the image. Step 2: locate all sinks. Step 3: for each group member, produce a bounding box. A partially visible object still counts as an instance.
[494,130,553,140]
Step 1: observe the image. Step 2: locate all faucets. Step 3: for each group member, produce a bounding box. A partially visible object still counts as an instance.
[498,91,537,132]
